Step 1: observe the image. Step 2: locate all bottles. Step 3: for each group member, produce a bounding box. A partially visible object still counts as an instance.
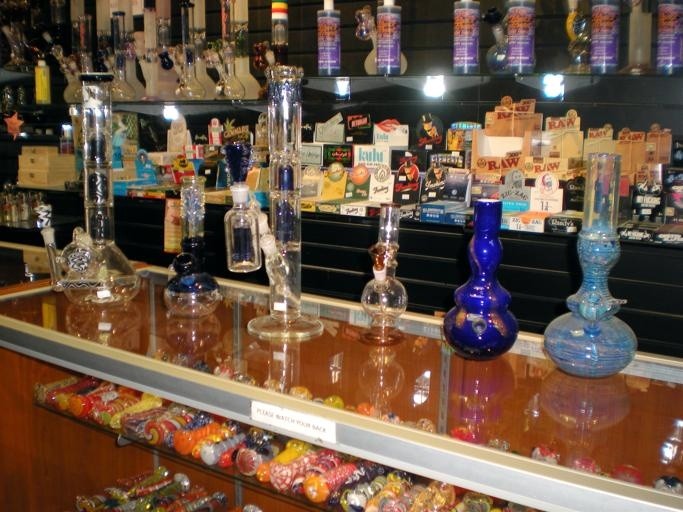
[621,0,656,74]
[537,367,632,460]
[62,300,144,352]
[317,0,342,78]
[226,183,264,272]
[0,179,48,230]
[446,352,515,445]
[41,228,64,292]
[507,0,535,75]
[358,204,409,347]
[375,0,402,76]
[164,319,224,378]
[270,0,289,66]
[566,0,592,74]
[56,71,142,313]
[483,7,536,75]
[452,0,480,75]
[544,153,639,379]
[163,172,221,317]
[35,16,247,105]
[590,1,620,74]
[358,345,406,420]
[245,64,326,341]
[265,343,301,392]
[354,5,407,76]
[440,198,521,359]
[656,0,683,75]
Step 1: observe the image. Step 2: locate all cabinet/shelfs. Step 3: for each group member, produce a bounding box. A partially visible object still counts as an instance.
[113,97,273,285]
[1,264,683,512]
[295,74,683,361]
[1,68,60,142]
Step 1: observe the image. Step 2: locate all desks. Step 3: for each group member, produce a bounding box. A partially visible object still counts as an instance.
[0,239,151,302]
[0,215,85,250]
